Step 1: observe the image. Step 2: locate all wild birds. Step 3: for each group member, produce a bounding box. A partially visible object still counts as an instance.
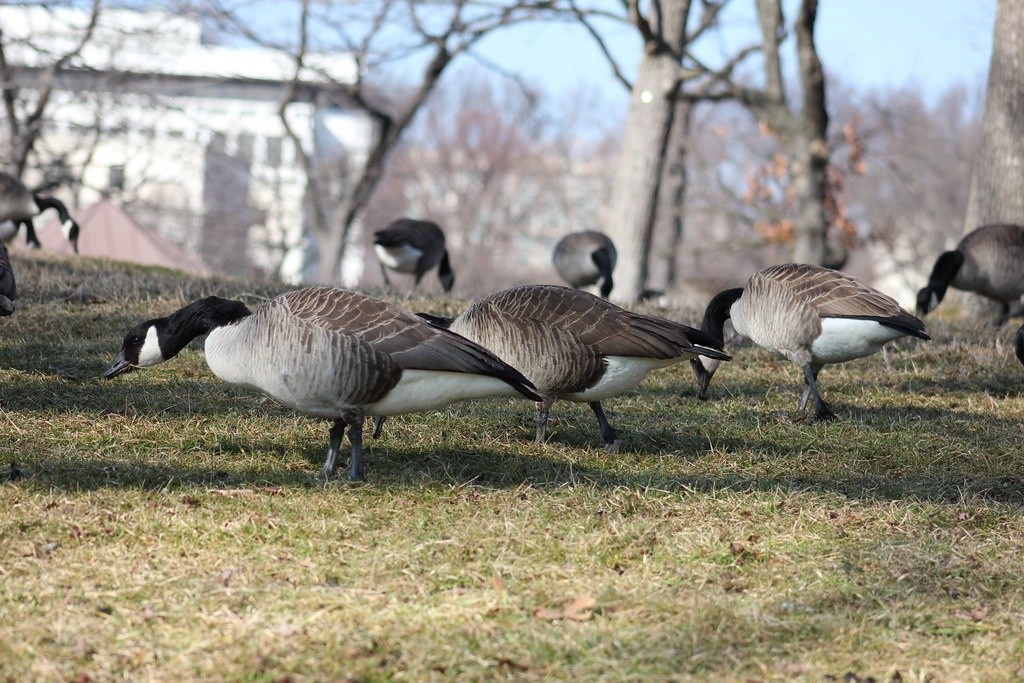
[688,263,932,424]
[372,284,735,453]
[553,232,617,301]
[0,171,80,316]
[102,286,543,487]
[373,218,455,301]
[915,223,1024,331]
